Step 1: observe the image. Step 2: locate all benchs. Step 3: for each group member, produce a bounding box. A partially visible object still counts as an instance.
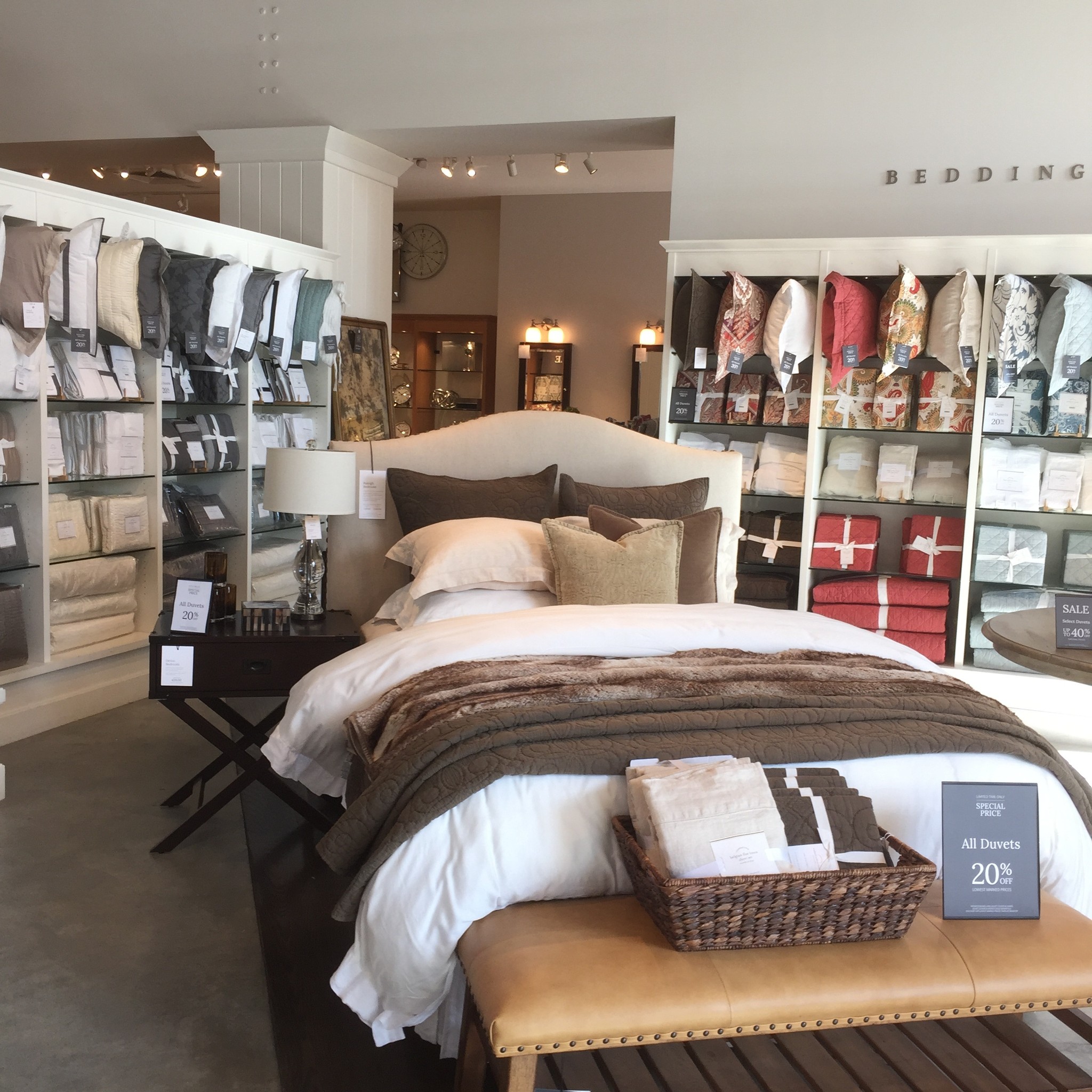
[456,880,1092,1092]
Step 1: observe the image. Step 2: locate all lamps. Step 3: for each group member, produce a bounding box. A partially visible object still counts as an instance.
[640,320,664,345]
[413,152,598,178]
[393,223,403,251]
[41,162,221,180]
[263,448,356,620]
[525,318,564,343]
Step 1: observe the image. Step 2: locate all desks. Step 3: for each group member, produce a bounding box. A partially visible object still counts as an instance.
[981,607,1092,686]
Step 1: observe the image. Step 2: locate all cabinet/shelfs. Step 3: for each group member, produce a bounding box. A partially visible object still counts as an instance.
[390,314,497,438]
[517,342,572,411]
[0,167,340,746]
[657,234,1092,679]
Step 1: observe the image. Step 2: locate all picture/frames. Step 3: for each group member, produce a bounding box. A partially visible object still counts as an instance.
[331,317,395,440]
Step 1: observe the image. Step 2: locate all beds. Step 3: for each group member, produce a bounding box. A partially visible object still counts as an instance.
[262,411,1092,1056]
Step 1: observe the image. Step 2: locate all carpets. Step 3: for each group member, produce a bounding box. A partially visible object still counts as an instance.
[0,698,281,1092]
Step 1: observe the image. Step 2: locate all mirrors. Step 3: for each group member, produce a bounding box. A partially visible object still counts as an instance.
[630,344,663,420]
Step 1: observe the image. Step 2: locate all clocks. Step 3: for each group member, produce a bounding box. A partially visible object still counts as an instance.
[400,224,448,280]
[390,346,412,438]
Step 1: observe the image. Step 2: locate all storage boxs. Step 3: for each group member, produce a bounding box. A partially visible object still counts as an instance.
[676,369,1090,436]
[241,600,291,631]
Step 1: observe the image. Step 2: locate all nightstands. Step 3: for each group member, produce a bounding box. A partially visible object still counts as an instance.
[149,610,360,852]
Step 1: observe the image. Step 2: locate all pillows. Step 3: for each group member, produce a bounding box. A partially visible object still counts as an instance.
[372,465,722,632]
[1,204,344,372]
[671,260,1092,398]
